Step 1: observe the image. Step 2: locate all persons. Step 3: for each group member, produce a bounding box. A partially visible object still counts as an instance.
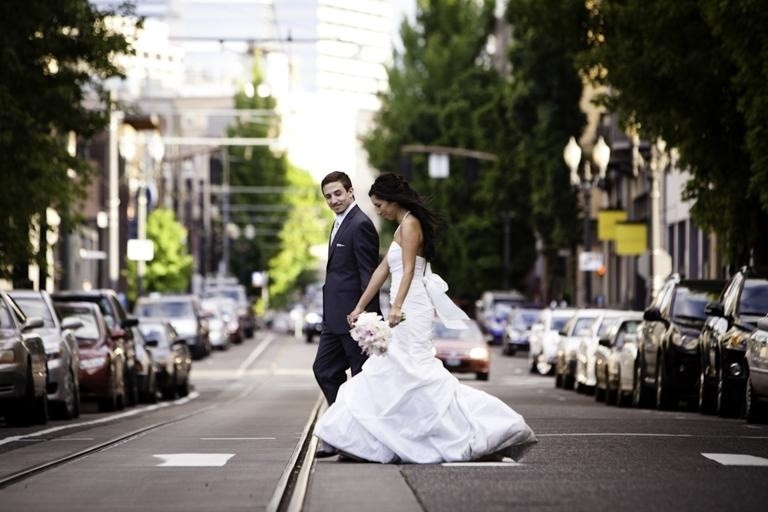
[312,171,385,462]
[312,173,539,464]
[550,291,569,307]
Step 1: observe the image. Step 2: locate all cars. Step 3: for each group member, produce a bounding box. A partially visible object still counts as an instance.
[431,318,494,382]
[742,307,768,425]
[304,309,324,341]
[475,290,644,407]
[0,279,256,425]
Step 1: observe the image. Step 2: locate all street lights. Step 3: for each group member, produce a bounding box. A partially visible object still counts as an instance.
[118,126,163,297]
[562,135,614,309]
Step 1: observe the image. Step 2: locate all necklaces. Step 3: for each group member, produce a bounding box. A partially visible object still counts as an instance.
[393,211,411,237]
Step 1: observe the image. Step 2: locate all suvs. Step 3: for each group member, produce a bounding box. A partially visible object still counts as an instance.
[696,264,767,417]
[631,275,728,407]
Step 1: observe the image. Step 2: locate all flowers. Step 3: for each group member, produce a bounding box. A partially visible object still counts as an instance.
[349,312,406,356]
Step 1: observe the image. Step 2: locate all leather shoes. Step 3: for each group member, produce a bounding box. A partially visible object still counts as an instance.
[315,448,339,458]
